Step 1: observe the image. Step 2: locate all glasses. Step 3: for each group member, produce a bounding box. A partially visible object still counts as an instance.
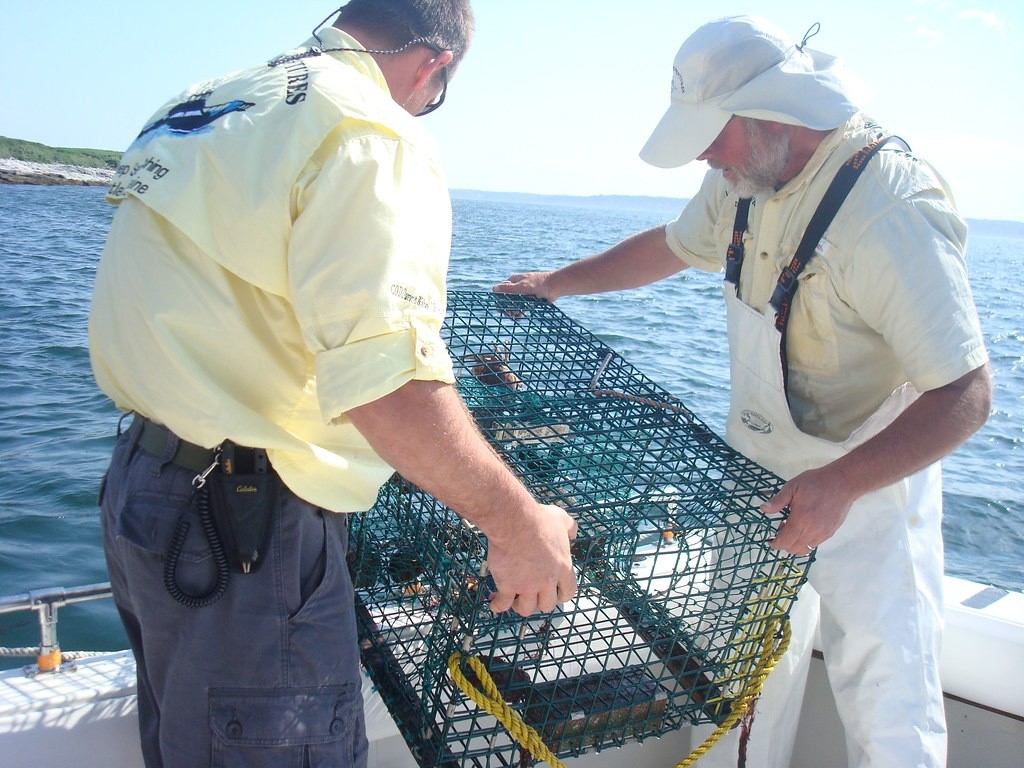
[422,41,450,114]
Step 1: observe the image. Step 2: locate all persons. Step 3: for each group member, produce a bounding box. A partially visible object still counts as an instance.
[87,0,578,768]
[494,20,991,768]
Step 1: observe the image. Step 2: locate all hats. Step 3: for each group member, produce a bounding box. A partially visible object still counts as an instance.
[639,14,874,168]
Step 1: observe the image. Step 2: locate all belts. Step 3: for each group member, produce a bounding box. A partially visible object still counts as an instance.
[123,408,271,477]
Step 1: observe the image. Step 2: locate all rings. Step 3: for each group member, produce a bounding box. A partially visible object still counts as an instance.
[807,544,816,552]
